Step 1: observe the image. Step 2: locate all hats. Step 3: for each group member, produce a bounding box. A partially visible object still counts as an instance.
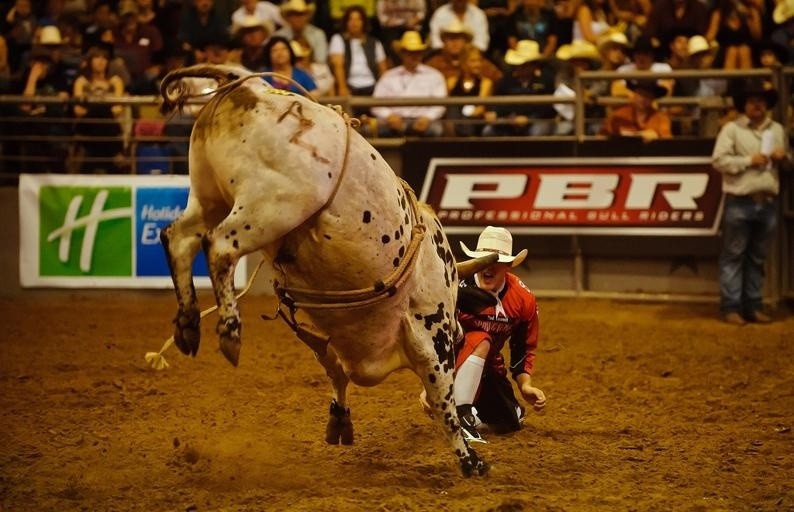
[393,31,429,54]
[731,82,780,114]
[684,36,720,58]
[439,22,475,45]
[228,1,316,58]
[624,76,667,96]
[32,27,70,46]
[505,31,671,66]
[460,226,528,269]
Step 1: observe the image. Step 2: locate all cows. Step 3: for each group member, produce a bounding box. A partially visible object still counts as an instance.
[158,62,499,478]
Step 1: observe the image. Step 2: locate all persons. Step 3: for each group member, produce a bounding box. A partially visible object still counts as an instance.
[0,0,792,187]
[420,225,546,446]
[709,83,792,326]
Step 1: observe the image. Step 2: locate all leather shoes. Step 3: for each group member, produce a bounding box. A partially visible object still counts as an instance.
[721,312,745,325]
[745,310,771,322]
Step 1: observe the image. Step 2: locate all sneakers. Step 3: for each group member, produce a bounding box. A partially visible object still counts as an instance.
[455,405,485,445]
